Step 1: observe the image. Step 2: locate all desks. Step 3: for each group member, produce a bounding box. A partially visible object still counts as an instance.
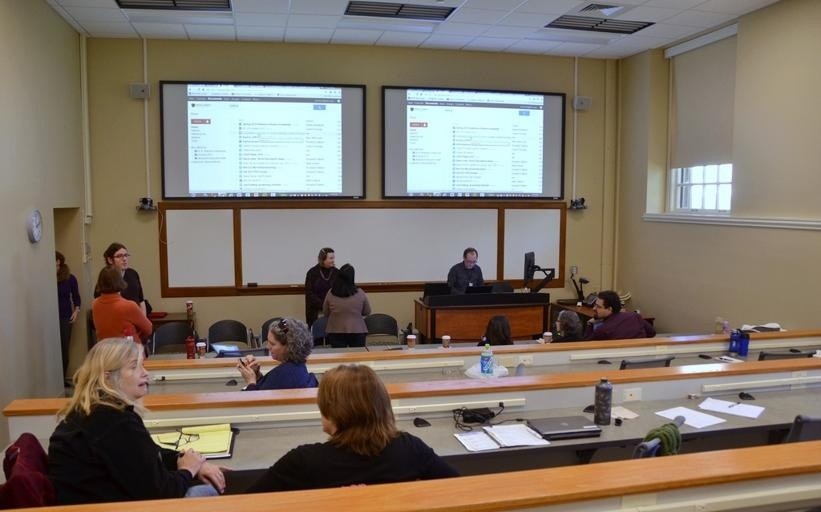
[551,299,656,341]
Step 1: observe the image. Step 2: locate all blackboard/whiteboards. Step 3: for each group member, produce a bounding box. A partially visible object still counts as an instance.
[157,200,567,299]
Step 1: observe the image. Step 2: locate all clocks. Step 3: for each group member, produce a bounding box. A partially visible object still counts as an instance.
[26,208,42,242]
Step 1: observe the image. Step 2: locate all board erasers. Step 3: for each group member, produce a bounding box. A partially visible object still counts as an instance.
[247,282,258,288]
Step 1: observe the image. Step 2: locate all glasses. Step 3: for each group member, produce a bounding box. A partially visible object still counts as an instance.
[278,318,289,334]
[157,430,199,450]
[114,254,130,257]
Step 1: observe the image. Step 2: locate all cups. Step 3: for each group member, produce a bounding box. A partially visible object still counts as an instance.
[184,300,194,315]
[441,334,452,349]
[407,334,417,351]
[196,341,207,359]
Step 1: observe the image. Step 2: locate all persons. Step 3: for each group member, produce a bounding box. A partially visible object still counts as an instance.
[236,361,469,493]
[56,250,82,390]
[324,262,373,348]
[551,308,585,342]
[478,314,516,347]
[94,241,150,359]
[238,316,319,391]
[46,337,228,502]
[91,264,153,347]
[304,245,340,347]
[446,245,486,299]
[588,289,659,340]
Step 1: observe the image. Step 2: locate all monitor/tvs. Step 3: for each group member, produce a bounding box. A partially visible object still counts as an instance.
[523,251,535,284]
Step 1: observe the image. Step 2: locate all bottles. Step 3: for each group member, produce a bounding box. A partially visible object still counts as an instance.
[728,329,742,358]
[478,343,496,381]
[740,330,751,357]
[594,376,613,427]
[185,334,196,359]
[543,331,553,346]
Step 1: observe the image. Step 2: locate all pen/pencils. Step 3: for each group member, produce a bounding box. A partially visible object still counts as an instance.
[719,357,733,362]
[384,347,403,352]
[728,401,740,408]
